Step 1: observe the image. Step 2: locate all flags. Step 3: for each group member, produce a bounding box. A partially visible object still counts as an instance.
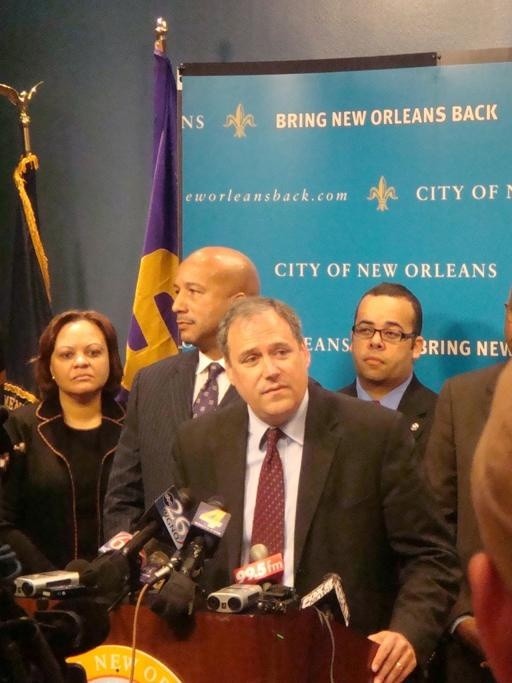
[3,154,54,410]
[112,54,180,409]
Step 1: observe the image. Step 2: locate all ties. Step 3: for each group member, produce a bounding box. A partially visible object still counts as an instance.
[245,427,287,586]
[189,361,226,420]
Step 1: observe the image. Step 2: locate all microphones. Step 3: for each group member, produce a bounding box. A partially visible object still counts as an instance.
[250,543,273,591]
[119,549,173,600]
[146,549,183,585]
[122,486,198,554]
[322,571,345,622]
[177,492,232,577]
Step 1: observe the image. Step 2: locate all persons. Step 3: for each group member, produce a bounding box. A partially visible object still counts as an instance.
[0,308,128,571]
[144,295,464,682]
[102,245,259,556]
[335,282,438,458]
[424,288,511,682]
[465,357,512,682]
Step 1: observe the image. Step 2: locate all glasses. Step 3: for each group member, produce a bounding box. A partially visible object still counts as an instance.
[349,326,417,343]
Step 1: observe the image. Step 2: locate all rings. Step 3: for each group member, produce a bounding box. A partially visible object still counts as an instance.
[394,659,405,670]
